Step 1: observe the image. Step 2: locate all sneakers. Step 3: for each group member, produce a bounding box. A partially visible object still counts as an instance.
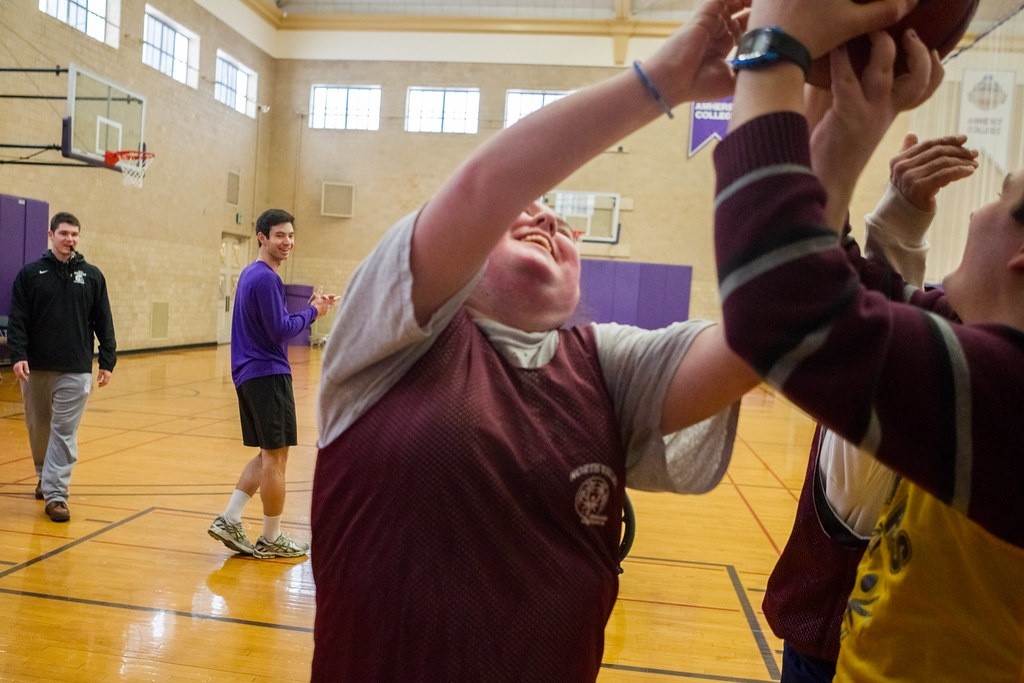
[253,532,309,559]
[208,514,254,554]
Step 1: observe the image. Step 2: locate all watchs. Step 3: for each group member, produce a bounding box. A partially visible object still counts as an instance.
[731,29,813,76]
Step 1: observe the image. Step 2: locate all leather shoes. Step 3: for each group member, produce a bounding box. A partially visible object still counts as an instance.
[45,501,69,522]
[35,480,43,499]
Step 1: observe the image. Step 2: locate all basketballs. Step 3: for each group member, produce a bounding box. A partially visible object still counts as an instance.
[806,0,979,92]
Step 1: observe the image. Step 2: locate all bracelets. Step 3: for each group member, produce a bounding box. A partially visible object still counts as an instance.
[632,58,675,120]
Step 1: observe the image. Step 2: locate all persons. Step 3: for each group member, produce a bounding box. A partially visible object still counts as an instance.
[310,2,1024,683]
[206,208,340,561]
[6,212,117,521]
